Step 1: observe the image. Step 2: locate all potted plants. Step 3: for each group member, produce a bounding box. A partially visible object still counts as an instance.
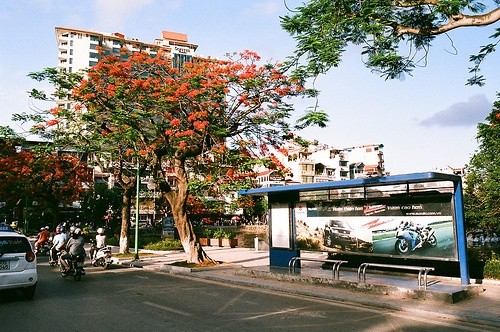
[199,230,210,245]
[222,231,238,247]
[209,230,221,245]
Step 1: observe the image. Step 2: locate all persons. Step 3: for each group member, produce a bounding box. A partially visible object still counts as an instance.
[33,223,85,274]
[395,219,423,252]
[91,227,107,264]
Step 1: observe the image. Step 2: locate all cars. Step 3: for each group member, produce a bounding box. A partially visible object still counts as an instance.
[0,221,37,298]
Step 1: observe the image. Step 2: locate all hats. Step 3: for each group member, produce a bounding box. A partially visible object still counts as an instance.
[40,228,45,230]
[45,226,49,229]
[74,228,81,234]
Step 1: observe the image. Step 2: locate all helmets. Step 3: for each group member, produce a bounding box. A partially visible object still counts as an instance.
[56,227,60,233]
[97,228,104,234]
[70,226,77,231]
[59,227,66,234]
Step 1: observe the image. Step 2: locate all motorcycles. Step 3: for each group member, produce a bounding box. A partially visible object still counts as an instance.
[395,225,437,255]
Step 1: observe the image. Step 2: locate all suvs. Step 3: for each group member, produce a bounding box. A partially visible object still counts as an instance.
[324,220,358,251]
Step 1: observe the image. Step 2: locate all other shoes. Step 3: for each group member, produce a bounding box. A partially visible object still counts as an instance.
[66,267,70,272]
[61,270,65,273]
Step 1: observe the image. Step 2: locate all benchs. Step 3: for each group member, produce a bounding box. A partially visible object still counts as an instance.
[358,263,434,290]
[288,256,348,279]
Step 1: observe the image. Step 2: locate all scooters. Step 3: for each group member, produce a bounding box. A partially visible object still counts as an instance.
[34,236,87,281]
[88,239,114,270]
